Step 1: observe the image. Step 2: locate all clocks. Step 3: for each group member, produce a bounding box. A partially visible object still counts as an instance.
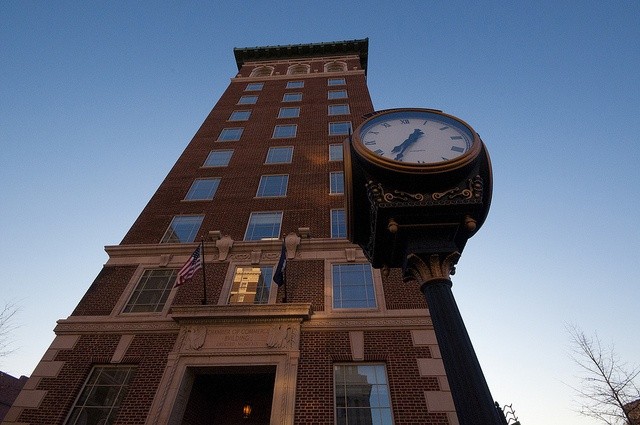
[346,107,493,269]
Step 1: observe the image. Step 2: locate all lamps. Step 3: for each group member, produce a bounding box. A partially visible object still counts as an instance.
[241,401,254,422]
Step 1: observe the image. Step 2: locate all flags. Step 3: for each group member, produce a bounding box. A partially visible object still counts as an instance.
[272,238,287,287]
[174,242,202,288]
